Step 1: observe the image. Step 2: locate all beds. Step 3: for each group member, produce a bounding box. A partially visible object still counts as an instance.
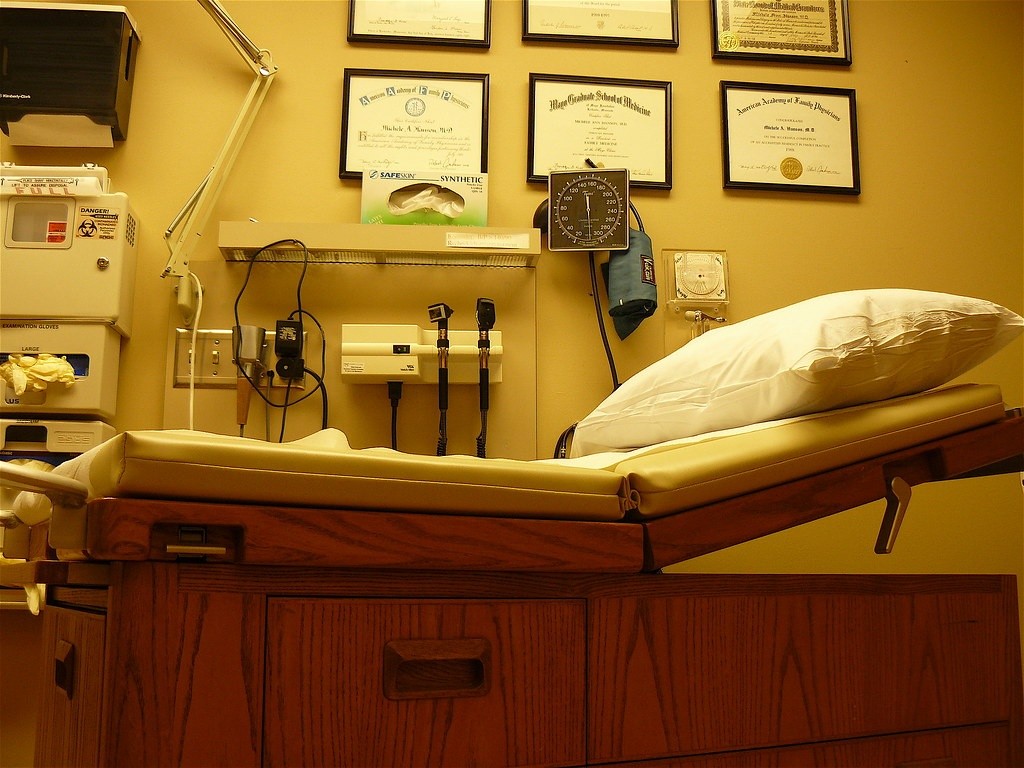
[46,382,1024,571]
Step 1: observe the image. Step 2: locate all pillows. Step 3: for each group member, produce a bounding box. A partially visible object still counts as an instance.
[568,288,1024,460]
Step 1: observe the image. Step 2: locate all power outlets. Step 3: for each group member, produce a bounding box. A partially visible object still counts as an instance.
[252,330,310,393]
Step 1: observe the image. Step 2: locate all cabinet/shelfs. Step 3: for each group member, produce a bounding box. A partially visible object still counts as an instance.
[32,558,1024,768]
